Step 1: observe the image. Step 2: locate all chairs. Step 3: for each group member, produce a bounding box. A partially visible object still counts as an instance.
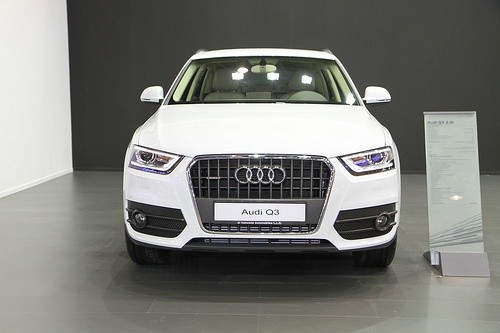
[278,69,315,100]
[204,69,245,99]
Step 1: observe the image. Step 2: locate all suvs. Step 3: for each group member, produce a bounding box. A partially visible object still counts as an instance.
[121,47,402,268]
